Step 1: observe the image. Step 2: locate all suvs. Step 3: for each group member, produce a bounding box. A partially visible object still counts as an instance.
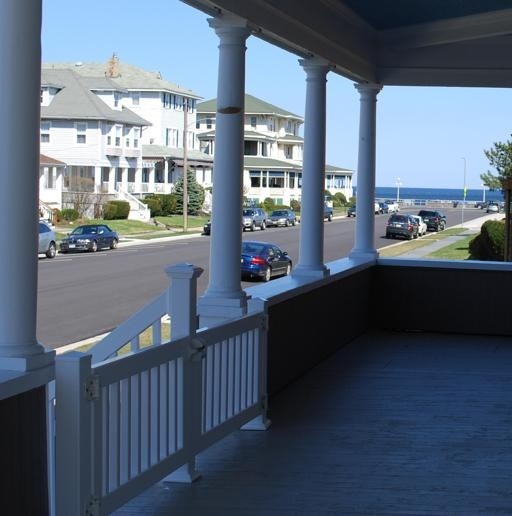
[385,213,420,240]
[418,209,447,232]
[243,207,269,232]
[38,218,58,259]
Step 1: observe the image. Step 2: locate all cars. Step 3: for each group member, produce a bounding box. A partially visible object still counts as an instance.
[240,240,294,284]
[204,219,211,234]
[410,214,428,236]
[323,202,334,222]
[266,209,298,227]
[486,201,501,214]
[473,199,503,209]
[60,223,121,255]
[346,204,357,217]
[375,198,401,216]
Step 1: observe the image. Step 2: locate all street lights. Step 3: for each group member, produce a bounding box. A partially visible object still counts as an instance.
[457,157,467,205]
[480,183,485,202]
[182,105,217,231]
[394,176,403,203]
